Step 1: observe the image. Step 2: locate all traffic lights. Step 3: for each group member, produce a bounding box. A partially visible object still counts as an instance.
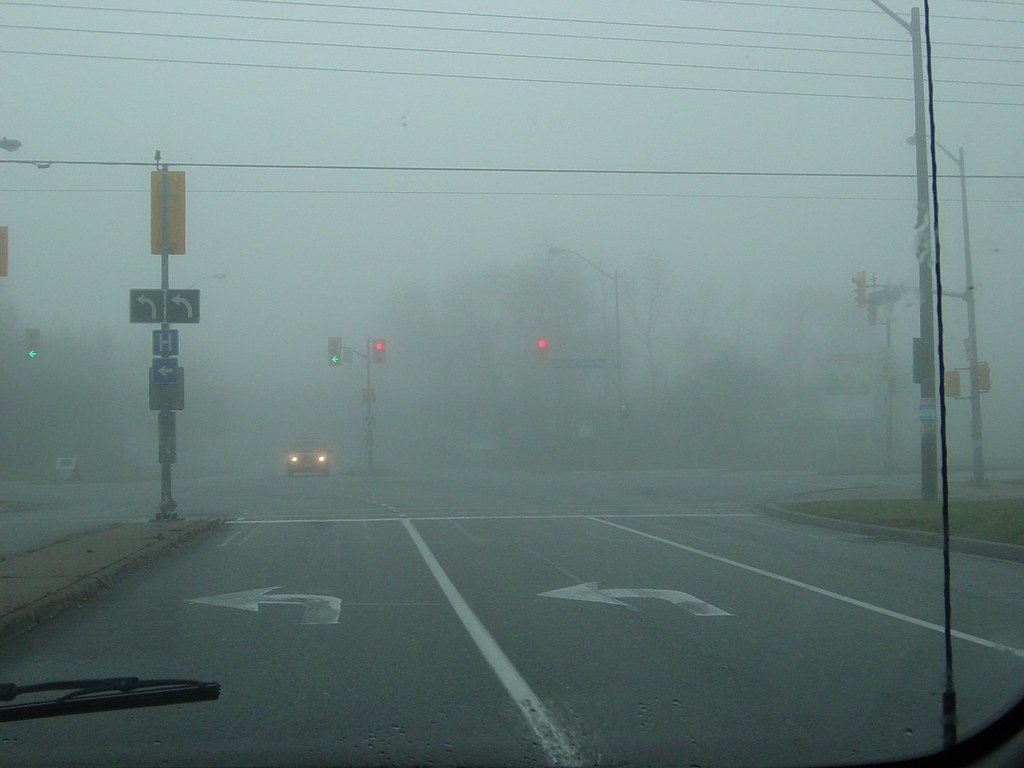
[371,337,386,365]
[328,336,344,366]
[973,363,990,389]
[852,270,866,305]
[24,328,40,366]
[867,299,877,323]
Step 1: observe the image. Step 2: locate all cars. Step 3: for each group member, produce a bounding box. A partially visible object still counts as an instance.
[283,436,334,477]
[54,456,87,482]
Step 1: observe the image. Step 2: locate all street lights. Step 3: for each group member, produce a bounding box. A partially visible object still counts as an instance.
[550,247,623,416]
[907,131,990,485]
